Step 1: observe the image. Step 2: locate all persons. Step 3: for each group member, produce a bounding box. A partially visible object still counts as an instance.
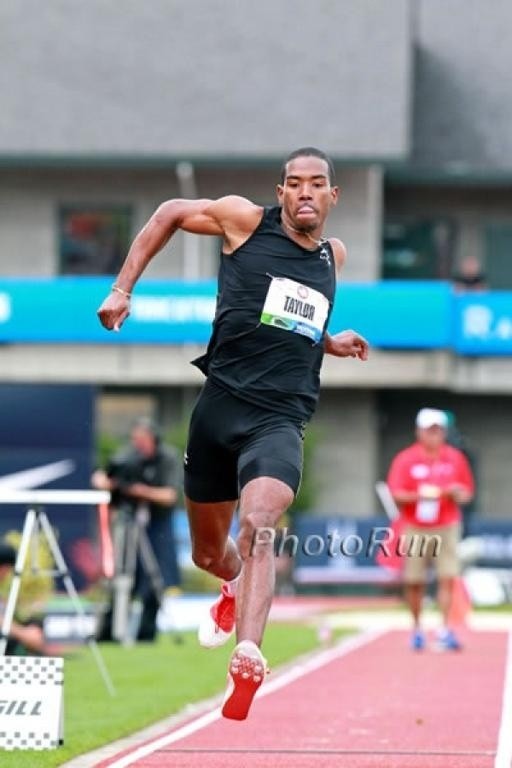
[97,146,369,721]
[387,407,474,651]
[0,546,68,657]
[92,420,183,641]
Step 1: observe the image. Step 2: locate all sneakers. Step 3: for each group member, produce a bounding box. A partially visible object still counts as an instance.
[198,584,237,647]
[222,639,270,720]
[412,631,459,649]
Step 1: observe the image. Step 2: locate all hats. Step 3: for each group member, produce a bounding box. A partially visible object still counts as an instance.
[417,408,448,429]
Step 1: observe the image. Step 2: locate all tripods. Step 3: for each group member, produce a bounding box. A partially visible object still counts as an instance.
[110,506,183,648]
[0,508,117,695]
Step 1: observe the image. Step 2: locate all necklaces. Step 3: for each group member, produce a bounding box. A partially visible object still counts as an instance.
[280,215,331,266]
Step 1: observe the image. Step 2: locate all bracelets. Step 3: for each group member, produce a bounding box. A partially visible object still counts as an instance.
[113,285,132,298]
